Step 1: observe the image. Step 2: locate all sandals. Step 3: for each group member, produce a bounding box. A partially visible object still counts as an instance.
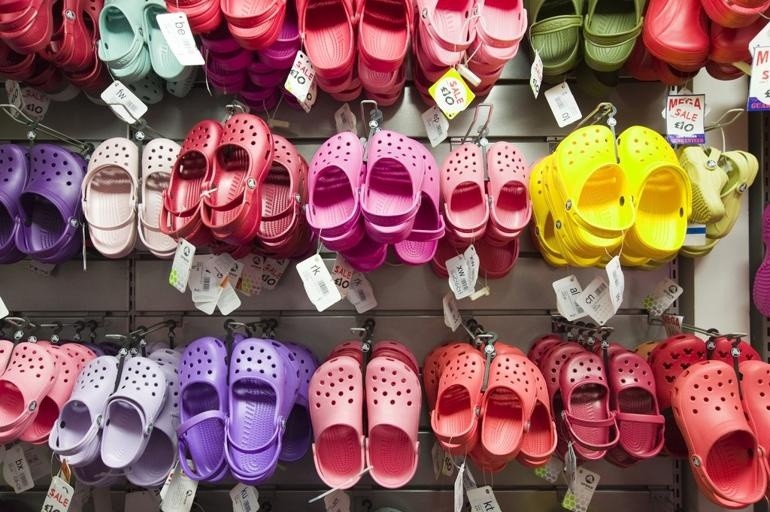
[295,0,418,109]
[47,337,184,496]
[308,338,425,490]
[0,139,87,267]
[156,113,315,265]
[431,137,533,280]
[0,1,301,116]
[527,332,667,469]
[419,340,559,476]
[634,332,770,512]
[78,132,192,261]
[177,334,318,486]
[409,0,529,110]
[677,140,759,260]
[622,0,770,89]
[520,0,649,102]
[527,121,696,270]
[305,127,448,274]
